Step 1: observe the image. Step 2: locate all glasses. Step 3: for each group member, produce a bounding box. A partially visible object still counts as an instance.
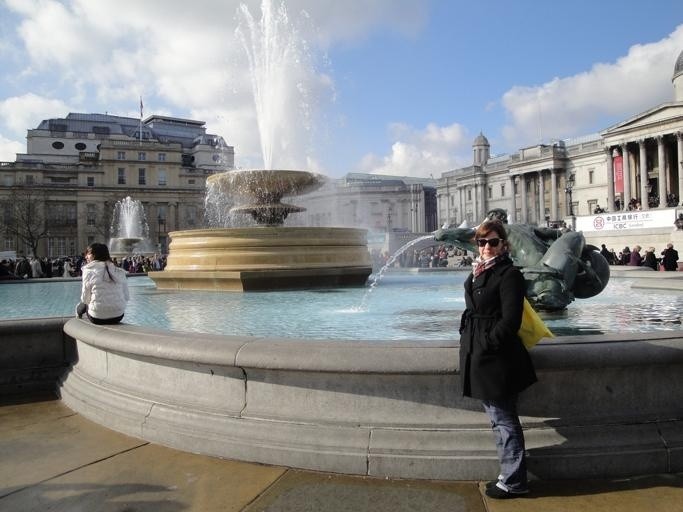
[474,236,503,248]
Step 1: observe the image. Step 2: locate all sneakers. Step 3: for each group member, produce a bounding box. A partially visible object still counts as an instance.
[478,478,501,488]
[478,485,525,499]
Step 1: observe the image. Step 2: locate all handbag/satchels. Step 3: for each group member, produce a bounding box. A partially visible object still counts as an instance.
[516,295,558,351]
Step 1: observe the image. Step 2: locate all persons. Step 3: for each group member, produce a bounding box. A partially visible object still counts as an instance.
[593,192,678,215]
[74,242,131,326]
[597,242,680,272]
[367,242,473,269]
[456,219,540,499]
[0,252,167,280]
